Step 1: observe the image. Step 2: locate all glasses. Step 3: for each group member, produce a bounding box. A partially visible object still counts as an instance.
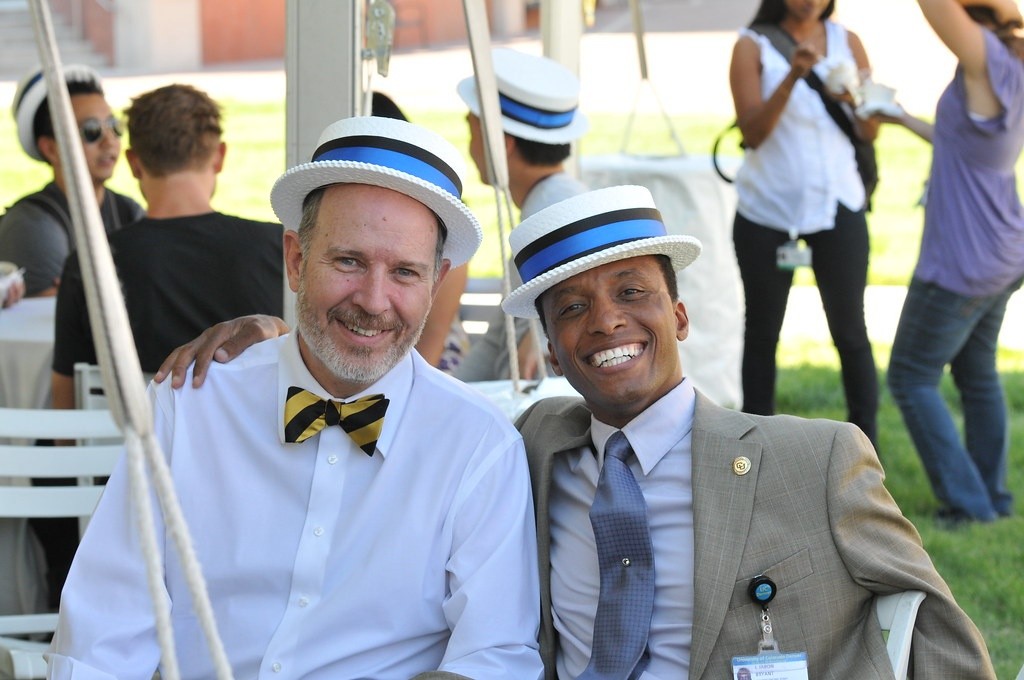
[79,116,126,144]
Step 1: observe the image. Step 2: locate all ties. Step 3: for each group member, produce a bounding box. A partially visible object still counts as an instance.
[574,432,656,679]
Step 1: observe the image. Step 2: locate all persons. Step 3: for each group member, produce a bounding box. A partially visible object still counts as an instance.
[153,182,998,680]
[872,0,1024,521]
[0,66,147,296]
[40,117,545,680]
[444,43,590,382]
[25,85,287,611]
[728,0,882,451]
[737,668,751,680]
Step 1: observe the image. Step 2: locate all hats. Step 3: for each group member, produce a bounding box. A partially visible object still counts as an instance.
[11,64,103,162]
[456,47,591,146]
[500,185,703,319]
[268,115,483,272]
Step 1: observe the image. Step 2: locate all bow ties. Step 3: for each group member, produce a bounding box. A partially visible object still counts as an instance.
[282,385,390,458]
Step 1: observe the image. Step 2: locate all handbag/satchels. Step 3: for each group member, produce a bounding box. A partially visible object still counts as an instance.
[846,121,878,211]
